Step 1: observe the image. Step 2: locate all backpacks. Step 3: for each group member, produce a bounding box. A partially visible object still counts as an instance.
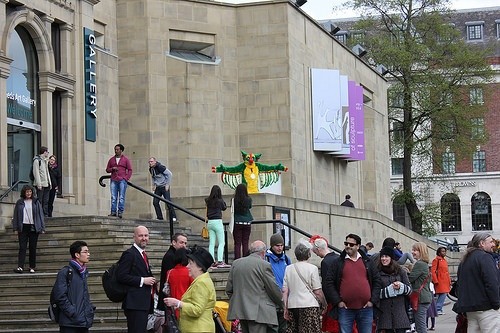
[48,266,72,323]
[102,249,136,303]
[30,155,41,181]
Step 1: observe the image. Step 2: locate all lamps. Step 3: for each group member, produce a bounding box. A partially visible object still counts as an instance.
[324,20,340,35]
[376,64,389,76]
[352,43,367,58]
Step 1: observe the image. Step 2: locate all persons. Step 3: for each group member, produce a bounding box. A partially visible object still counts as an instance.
[148,157,177,221]
[444,237,453,252]
[452,231,500,333]
[225,234,439,333]
[204,184,231,268]
[13,185,45,274]
[32,146,59,218]
[156,232,216,333]
[106,144,132,218]
[116,225,159,333]
[228,183,254,260]
[453,237,460,252]
[52,241,96,333]
[341,194,355,208]
[430,246,451,315]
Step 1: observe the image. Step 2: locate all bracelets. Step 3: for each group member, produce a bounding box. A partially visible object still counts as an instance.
[284,307,287,310]
[177,300,181,308]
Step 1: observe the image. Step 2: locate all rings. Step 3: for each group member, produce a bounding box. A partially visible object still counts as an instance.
[167,303,168,305]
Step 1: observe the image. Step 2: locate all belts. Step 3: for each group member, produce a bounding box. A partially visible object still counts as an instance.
[235,222,251,224]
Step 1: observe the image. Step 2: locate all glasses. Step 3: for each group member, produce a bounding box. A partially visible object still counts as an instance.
[78,251,89,254]
[344,242,358,247]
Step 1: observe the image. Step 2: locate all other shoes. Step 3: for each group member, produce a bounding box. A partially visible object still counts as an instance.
[30,268,35,273]
[437,311,445,315]
[209,262,232,268]
[173,218,176,221]
[108,214,122,219]
[18,267,23,271]
[429,328,434,331]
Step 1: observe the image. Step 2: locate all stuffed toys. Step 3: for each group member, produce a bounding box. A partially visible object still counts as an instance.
[211,151,288,194]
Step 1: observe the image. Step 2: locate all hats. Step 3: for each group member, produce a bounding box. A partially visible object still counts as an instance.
[310,235,320,243]
[380,247,394,259]
[186,244,214,273]
[270,234,284,246]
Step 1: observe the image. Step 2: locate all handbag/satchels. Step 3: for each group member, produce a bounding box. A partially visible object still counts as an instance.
[229,198,235,234]
[161,304,178,333]
[31,225,36,231]
[162,269,173,296]
[202,227,209,238]
[409,291,418,311]
[449,282,458,298]
[212,311,227,333]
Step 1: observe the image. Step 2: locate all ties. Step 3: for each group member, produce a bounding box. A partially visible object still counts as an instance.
[142,251,154,299]
